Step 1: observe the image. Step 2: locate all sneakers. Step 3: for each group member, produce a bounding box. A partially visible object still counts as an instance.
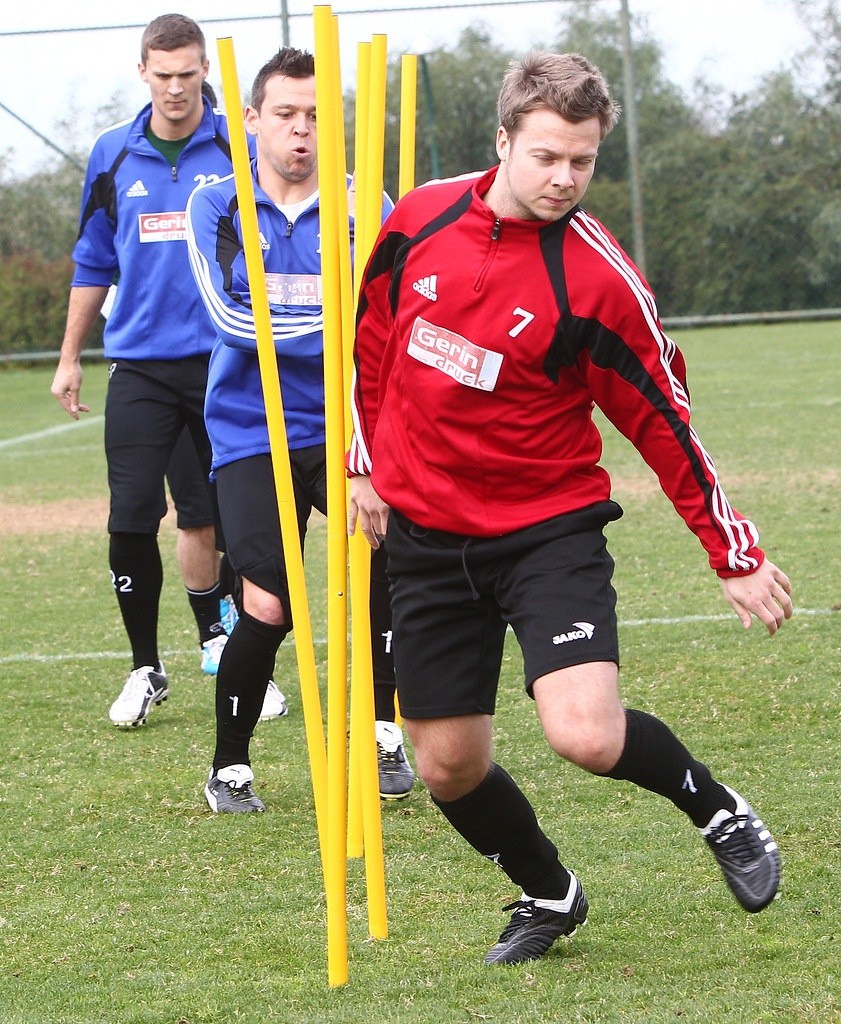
[219,595,239,635]
[205,764,266,814]
[483,870,589,968]
[109,659,169,726]
[375,721,414,801]
[258,680,288,721]
[696,782,782,913]
[200,635,229,674]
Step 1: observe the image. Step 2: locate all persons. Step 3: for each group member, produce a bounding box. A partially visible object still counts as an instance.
[185,46,414,816]
[51,13,294,727]
[349,52,793,969]
